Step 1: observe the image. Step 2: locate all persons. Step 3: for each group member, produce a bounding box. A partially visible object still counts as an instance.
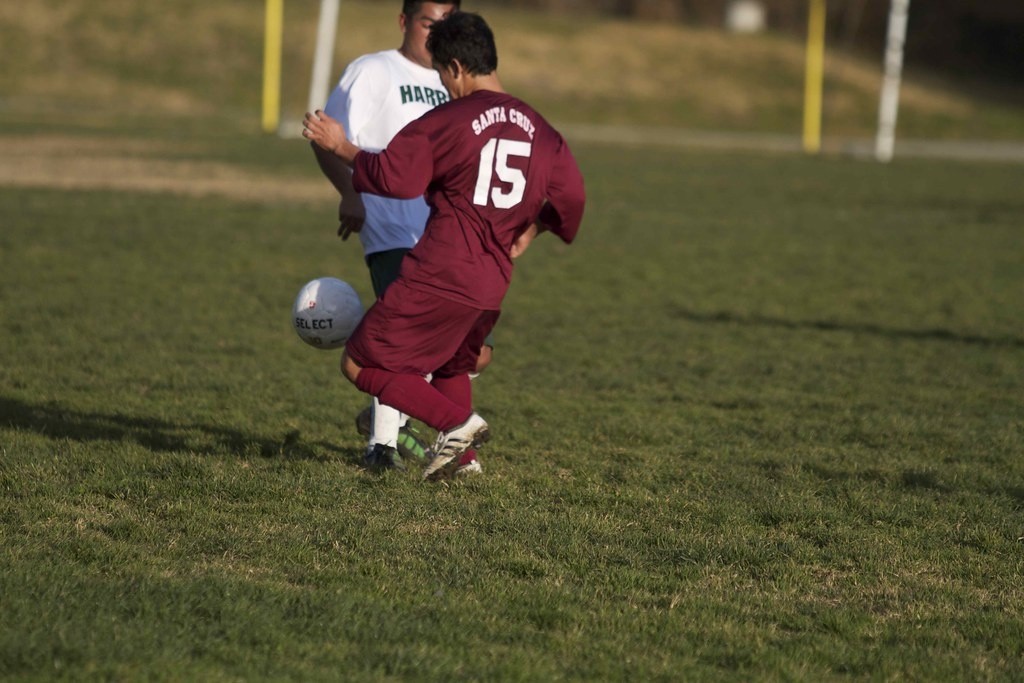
[309,0,464,471]
[302,13,586,483]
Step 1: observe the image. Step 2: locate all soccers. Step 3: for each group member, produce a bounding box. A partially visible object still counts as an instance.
[293,275,364,350]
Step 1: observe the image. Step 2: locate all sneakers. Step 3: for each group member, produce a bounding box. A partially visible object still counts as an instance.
[359,440,409,477]
[423,443,483,476]
[419,412,490,486]
[356,407,435,468]
